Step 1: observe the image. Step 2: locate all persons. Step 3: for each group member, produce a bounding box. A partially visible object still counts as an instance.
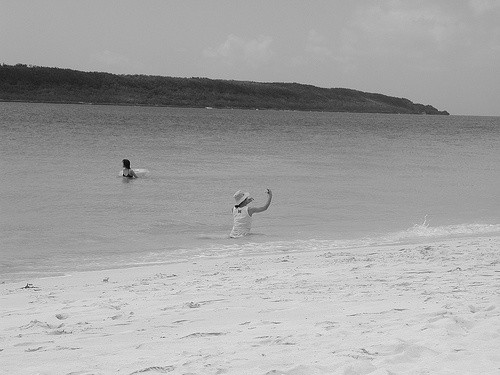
[120,158,138,178]
[229,188,272,238]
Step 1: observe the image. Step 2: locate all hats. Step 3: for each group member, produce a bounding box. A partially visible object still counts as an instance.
[233,190,249,206]
